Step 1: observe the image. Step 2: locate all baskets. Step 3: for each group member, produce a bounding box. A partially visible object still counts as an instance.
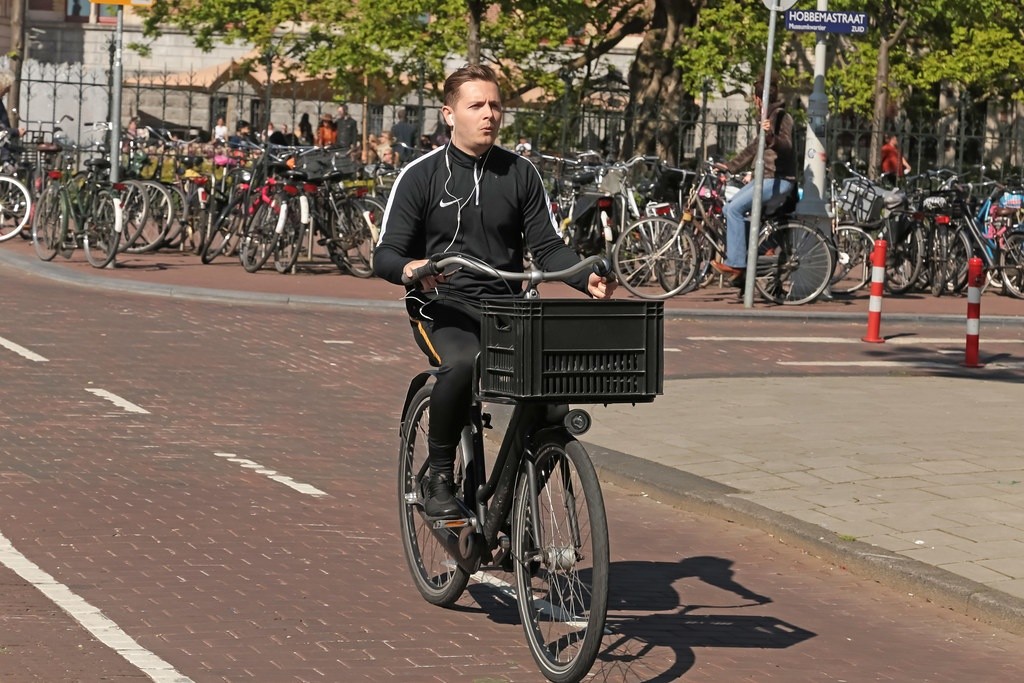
[296,147,356,181]
[22,128,54,164]
[479,297,664,402]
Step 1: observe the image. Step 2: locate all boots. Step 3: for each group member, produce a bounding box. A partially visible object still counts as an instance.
[422,439,461,521]
[502,531,540,577]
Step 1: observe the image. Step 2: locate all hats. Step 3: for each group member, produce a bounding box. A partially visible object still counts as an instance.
[322,114,334,123]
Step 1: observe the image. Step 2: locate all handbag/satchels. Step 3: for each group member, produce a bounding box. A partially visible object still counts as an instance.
[652,167,696,204]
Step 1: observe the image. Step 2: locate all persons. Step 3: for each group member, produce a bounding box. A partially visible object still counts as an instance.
[210,103,453,171]
[876,131,912,178]
[369,60,619,578]
[119,115,141,154]
[0,66,27,220]
[711,80,800,288]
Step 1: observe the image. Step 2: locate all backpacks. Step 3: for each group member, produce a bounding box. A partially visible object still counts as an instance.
[774,110,806,170]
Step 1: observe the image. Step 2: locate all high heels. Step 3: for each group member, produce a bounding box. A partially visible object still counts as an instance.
[710,260,743,286]
[765,248,775,255]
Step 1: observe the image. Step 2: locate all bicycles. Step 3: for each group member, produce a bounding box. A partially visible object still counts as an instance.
[394,251,667,682]
[0,110,1024,299]
